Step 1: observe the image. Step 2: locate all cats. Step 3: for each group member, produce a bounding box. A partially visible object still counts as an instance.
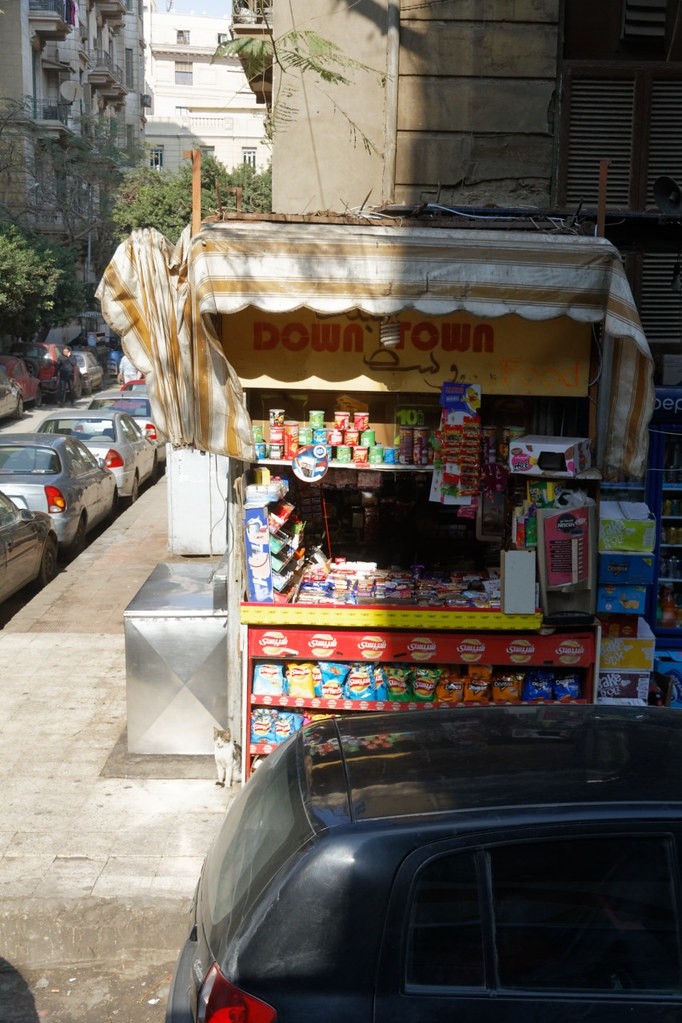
[213,727,242,788]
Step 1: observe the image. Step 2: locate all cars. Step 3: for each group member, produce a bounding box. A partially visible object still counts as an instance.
[34,410,157,504]
[0,343,104,419]
[0,432,118,557]
[0,489,58,604]
[86,391,165,475]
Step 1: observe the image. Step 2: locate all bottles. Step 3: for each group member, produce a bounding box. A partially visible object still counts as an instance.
[656,497,682,629]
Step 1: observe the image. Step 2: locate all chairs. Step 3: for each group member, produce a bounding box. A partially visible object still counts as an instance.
[103,428,114,440]
[55,428,73,435]
[135,407,146,415]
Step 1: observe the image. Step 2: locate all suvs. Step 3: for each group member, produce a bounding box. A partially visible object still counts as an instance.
[120,380,147,391]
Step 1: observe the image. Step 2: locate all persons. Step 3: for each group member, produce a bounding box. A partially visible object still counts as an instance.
[53,347,77,407]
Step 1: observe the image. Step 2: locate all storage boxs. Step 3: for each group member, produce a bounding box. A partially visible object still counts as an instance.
[509,434,593,476]
[596,584,646,615]
[599,512,657,552]
[597,551,656,584]
[597,671,651,706]
[600,615,657,671]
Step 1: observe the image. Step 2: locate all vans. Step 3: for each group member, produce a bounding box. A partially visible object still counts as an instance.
[165,705,682,1023]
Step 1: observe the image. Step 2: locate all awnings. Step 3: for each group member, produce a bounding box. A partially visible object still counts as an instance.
[93,219,654,479]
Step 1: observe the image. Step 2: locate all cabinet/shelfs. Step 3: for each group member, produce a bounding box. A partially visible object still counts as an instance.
[246,625,595,780]
